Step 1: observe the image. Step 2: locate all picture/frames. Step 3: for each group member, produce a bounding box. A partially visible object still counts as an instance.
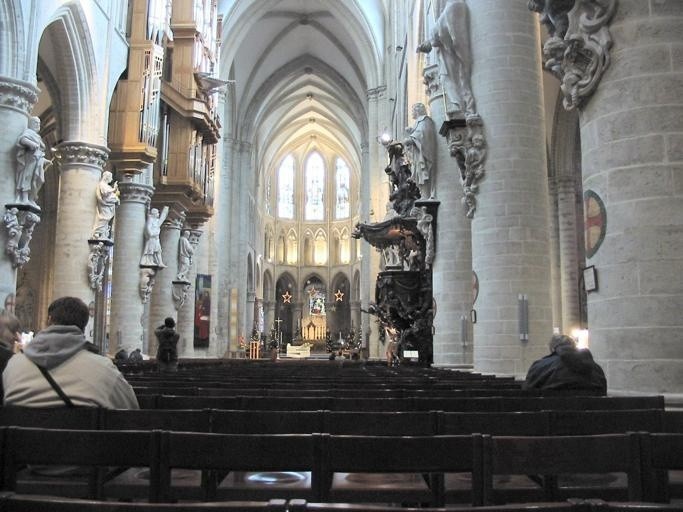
[581,264,598,293]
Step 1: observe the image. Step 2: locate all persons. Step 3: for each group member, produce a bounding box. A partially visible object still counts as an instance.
[335,351,345,368]
[16,116,52,210]
[270,343,278,360]
[415,0,474,119]
[328,352,336,359]
[142,208,167,267]
[153,317,179,374]
[128,348,142,364]
[2,297,140,477]
[520,334,607,397]
[3,207,19,228]
[177,231,194,280]
[0,308,20,407]
[115,349,128,365]
[402,103,436,200]
[90,171,120,238]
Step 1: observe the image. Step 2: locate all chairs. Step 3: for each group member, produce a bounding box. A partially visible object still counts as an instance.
[0,350,683,512]
